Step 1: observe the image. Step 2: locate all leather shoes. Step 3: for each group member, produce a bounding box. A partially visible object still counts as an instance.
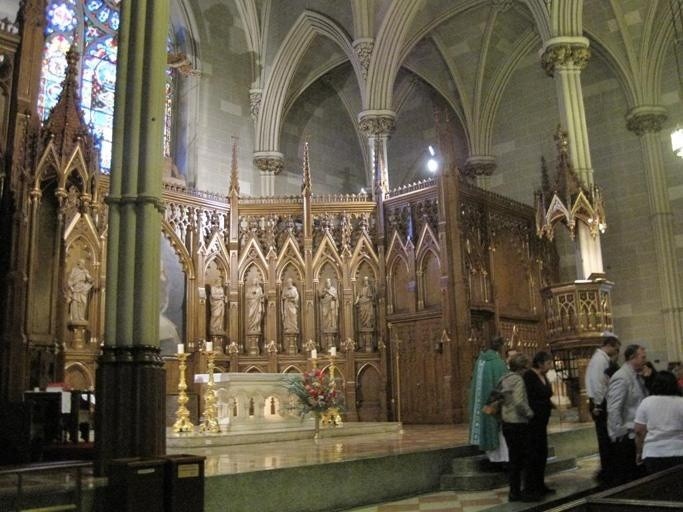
[510,486,555,502]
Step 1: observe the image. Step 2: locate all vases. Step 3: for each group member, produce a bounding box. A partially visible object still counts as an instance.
[312,407,323,438]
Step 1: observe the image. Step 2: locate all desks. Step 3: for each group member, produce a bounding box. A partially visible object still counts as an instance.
[0,459,94,512]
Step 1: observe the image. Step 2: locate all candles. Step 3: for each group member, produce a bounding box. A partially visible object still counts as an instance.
[311,349,317,359]
[330,346,336,356]
[177,344,184,354]
[206,341,213,351]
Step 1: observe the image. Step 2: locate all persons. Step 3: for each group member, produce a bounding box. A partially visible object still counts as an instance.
[281,278,299,334]
[68,259,95,320]
[245,279,264,332]
[318,279,339,331]
[355,276,374,329]
[209,278,226,336]
[585,338,683,476]
[466,336,556,503]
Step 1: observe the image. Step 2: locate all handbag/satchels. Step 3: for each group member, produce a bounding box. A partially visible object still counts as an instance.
[480,390,505,428]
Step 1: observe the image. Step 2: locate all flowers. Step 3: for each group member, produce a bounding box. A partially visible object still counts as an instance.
[280,365,344,423]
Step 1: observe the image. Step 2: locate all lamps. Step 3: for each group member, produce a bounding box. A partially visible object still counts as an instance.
[669,127,683,159]
